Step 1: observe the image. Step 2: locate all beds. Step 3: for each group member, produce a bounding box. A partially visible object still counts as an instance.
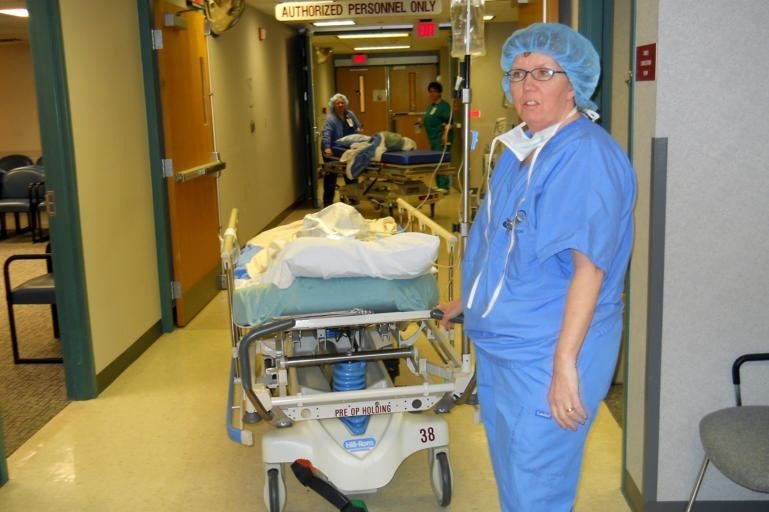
[317,139,458,219]
[220,197,479,512]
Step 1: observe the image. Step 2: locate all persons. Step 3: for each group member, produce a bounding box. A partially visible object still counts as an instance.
[430,21,639,512]
[321,93,363,208]
[418,81,453,194]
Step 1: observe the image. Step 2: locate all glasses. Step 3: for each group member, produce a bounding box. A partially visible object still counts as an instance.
[505,68,566,81]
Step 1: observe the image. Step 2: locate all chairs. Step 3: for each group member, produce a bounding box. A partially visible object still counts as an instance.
[0,153,65,364]
[687,352,769,512]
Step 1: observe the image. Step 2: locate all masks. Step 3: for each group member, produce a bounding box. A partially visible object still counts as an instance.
[496,125,556,162]
